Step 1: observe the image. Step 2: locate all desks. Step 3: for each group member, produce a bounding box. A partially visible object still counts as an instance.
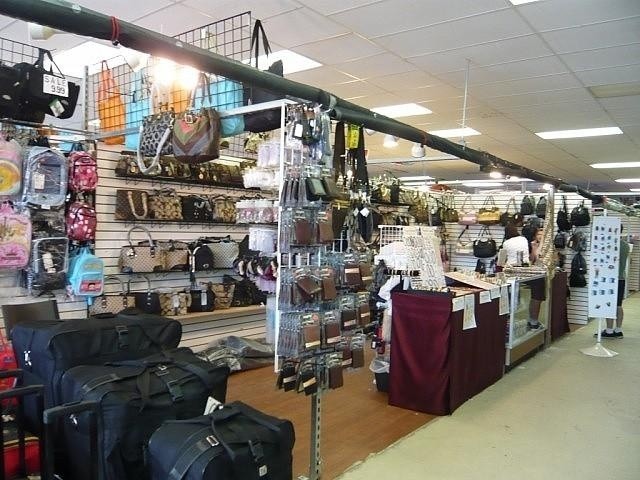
[500,271,552,367]
[389,282,507,417]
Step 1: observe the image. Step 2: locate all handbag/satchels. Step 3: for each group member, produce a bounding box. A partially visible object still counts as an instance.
[0,330,20,408]
[51,348,229,479]
[0,60,80,128]
[12,307,182,436]
[146,400,295,480]
[87,150,268,317]
[98,58,286,162]
[371,183,590,299]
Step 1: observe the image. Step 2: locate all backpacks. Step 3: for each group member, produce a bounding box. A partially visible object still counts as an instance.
[1,134,104,305]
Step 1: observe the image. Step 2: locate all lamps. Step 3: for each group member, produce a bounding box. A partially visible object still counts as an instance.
[27,23,72,41]
[411,135,425,158]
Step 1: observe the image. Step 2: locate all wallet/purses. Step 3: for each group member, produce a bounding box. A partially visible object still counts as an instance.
[275,177,373,396]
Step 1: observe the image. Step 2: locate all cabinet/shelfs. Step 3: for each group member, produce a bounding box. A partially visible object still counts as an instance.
[116,150,417,330]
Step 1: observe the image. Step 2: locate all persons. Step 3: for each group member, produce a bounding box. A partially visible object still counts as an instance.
[493,245,503,273]
[593,223,630,340]
[522,225,546,331]
[503,224,530,267]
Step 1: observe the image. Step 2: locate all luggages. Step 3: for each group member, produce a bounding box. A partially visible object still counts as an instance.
[0,369,63,480]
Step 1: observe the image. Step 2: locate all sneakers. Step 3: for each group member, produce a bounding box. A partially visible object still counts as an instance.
[594,330,616,339]
[615,331,622,338]
[528,321,542,329]
